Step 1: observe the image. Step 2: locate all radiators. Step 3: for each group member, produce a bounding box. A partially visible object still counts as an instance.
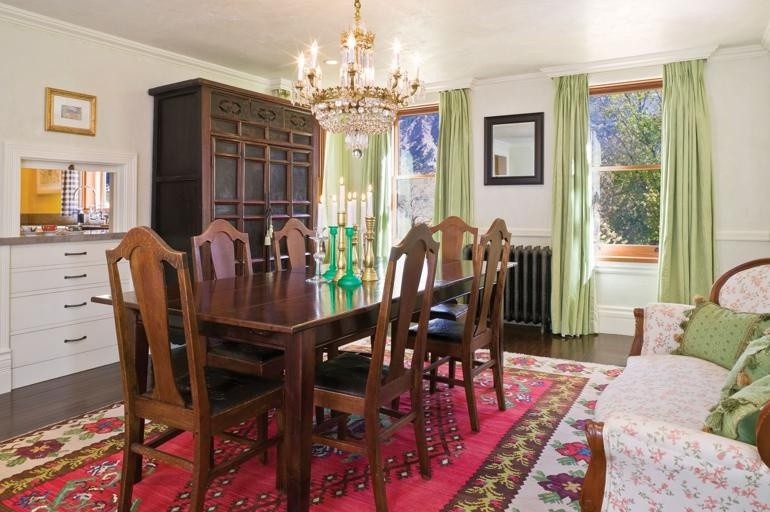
[460,240,552,340]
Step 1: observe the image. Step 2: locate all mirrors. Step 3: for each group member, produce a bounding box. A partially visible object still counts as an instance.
[482,111,545,186]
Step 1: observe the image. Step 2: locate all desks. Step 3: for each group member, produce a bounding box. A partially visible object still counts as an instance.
[91,261,517,512]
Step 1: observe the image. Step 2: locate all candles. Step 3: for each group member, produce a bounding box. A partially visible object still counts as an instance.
[330,185,373,229]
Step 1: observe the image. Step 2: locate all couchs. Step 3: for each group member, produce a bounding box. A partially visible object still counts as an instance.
[579,257,770,511]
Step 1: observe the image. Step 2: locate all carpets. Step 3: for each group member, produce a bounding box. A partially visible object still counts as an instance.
[1,335,626,512]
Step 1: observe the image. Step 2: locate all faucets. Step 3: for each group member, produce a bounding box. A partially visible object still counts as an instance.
[73,185,97,212]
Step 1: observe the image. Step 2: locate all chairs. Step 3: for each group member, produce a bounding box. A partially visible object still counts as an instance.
[387,218,513,435]
[270,217,351,446]
[186,219,288,465]
[108,225,287,512]
[390,213,478,392]
[302,224,444,512]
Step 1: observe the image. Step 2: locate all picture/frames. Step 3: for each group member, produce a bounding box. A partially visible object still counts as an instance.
[393,104,443,179]
[44,86,97,137]
[36,170,60,195]
[390,175,442,250]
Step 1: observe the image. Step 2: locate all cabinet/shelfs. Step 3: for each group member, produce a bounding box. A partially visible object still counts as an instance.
[9,234,144,394]
[267,99,322,243]
[150,85,267,251]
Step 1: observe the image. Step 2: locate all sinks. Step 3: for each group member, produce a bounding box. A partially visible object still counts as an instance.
[66,226,108,230]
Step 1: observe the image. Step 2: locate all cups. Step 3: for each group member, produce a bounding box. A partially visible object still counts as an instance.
[41,224,57,231]
[65,225,83,232]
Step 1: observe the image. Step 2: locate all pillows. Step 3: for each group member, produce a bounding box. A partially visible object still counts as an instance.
[668,292,770,370]
[708,334,769,412]
[702,376,769,445]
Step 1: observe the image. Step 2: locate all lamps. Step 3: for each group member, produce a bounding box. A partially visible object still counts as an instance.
[289,0,422,161]
